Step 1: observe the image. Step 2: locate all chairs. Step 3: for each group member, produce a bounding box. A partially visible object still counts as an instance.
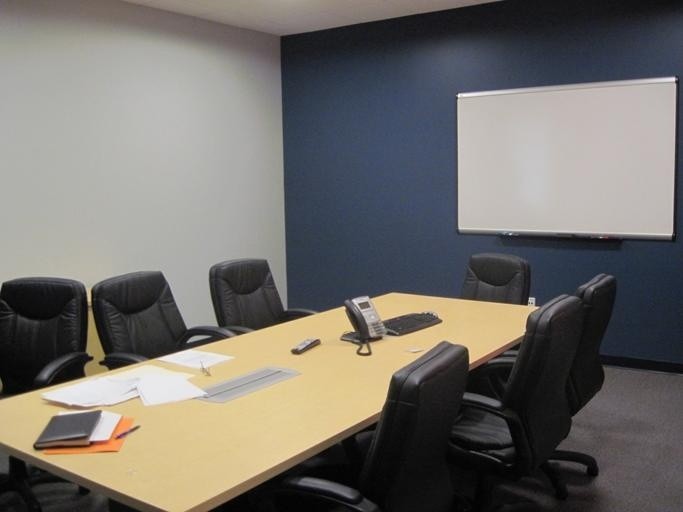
[3,276,94,396]
[441,291,581,510]
[266,339,469,512]
[457,253,531,303]
[209,258,317,334]
[471,274,620,496]
[89,269,235,371]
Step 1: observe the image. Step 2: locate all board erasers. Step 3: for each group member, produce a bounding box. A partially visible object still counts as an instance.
[501,232,519,239]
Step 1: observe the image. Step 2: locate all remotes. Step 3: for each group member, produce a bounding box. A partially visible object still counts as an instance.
[291,338,320,354]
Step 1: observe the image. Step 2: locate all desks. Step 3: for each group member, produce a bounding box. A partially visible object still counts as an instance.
[1,291,542,512]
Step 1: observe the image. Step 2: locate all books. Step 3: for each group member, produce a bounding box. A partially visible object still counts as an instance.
[33,410,104,448]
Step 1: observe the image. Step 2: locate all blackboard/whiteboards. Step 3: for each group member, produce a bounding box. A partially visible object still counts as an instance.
[455,76,679,243]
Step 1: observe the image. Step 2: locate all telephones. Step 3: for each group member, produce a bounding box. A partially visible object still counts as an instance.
[345,294,386,344]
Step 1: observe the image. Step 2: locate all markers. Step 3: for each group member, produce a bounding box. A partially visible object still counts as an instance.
[591,236,609,240]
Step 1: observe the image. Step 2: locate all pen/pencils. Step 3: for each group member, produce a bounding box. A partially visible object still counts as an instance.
[115,425,142,441]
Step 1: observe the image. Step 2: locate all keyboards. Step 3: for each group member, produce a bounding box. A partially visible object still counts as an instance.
[383,313,442,335]
